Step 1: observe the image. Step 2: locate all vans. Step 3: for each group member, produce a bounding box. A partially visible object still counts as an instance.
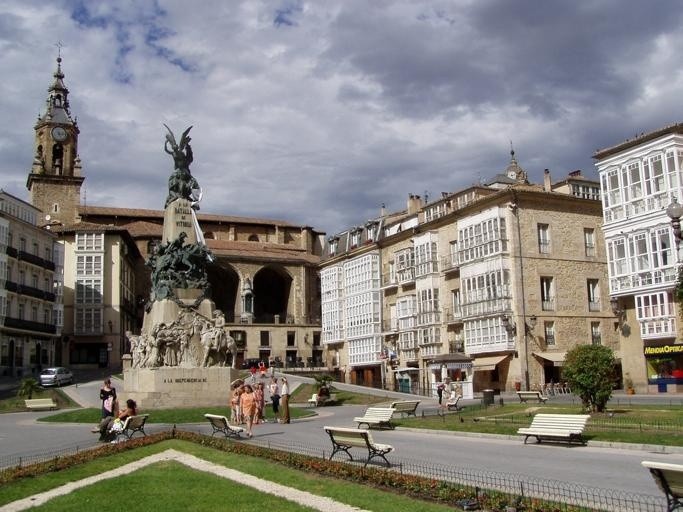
[242,358,268,369]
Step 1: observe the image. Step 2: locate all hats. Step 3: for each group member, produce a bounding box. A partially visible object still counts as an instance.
[103,378,110,385]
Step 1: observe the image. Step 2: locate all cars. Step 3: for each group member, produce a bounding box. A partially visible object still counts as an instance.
[39,367,73,387]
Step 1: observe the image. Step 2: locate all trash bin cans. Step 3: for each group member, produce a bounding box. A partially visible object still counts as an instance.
[397,378,409,393]
[659,381,667,392]
[484,389,494,405]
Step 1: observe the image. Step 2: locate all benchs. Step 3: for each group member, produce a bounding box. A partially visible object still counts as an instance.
[204,412,243,442]
[515,391,547,405]
[108,413,150,443]
[516,413,590,448]
[323,400,421,469]
[445,394,461,411]
[639,460,682,512]
[24,398,56,411]
[307,394,335,407]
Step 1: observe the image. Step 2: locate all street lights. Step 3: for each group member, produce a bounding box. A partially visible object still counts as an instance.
[667,196,682,263]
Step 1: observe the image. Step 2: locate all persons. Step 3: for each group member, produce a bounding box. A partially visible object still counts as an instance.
[248,364,256,382]
[434,375,462,410]
[164,138,192,197]
[99,378,116,417]
[257,358,267,376]
[315,381,330,407]
[124,231,238,369]
[230,374,291,438]
[90,398,137,442]
